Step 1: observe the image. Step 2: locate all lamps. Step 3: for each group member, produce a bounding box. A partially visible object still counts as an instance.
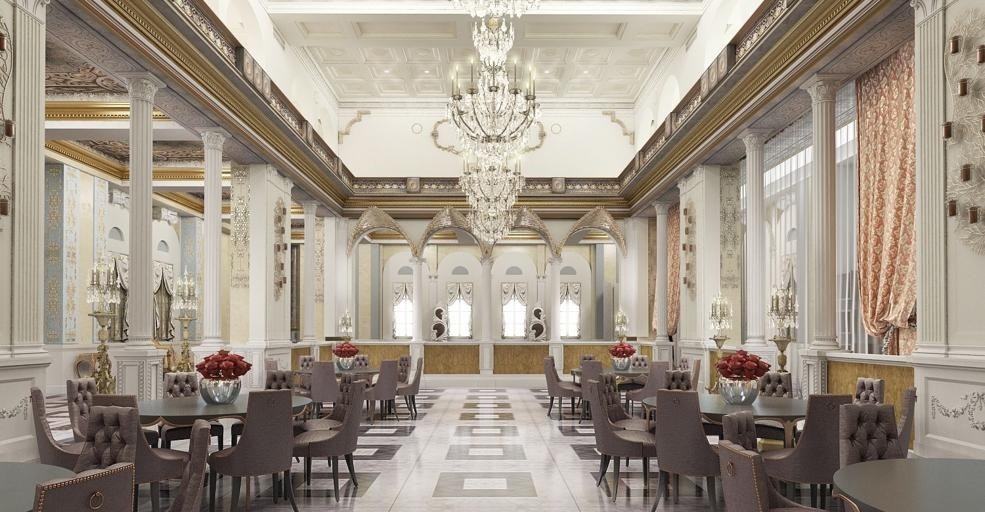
[338,309,353,340]
[443,1,545,243]
[175,267,198,372]
[615,309,628,341]
[709,296,732,359]
[85,256,120,395]
[767,276,798,375]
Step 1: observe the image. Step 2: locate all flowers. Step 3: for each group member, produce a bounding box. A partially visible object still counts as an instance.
[333,341,359,358]
[609,341,636,357]
